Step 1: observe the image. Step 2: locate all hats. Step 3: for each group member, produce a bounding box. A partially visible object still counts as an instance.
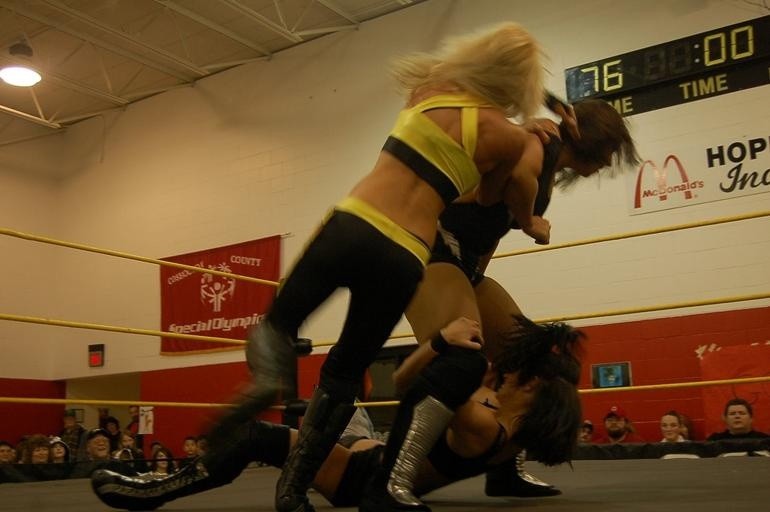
[86,428,109,441]
[50,437,68,451]
[603,404,625,419]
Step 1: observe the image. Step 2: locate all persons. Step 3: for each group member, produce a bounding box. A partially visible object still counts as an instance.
[575,397,769,447]
[216,20,581,512]
[89,313,582,510]
[2,404,210,480]
[364,97,645,505]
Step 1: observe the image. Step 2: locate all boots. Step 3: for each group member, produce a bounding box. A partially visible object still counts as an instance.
[376,386,456,512]
[91,458,231,511]
[271,386,358,511]
[485,445,561,497]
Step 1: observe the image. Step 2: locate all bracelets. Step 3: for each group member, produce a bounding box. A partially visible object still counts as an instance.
[423,326,448,356]
